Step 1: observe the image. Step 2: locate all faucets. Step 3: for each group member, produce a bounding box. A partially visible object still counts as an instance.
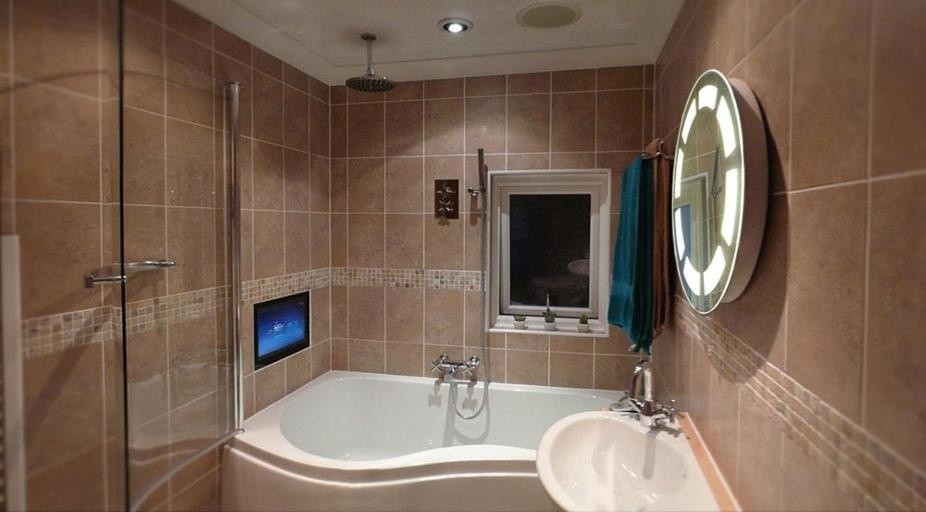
[627,359,668,429]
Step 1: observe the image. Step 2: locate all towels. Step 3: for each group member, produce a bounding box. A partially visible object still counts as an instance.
[608,138,671,356]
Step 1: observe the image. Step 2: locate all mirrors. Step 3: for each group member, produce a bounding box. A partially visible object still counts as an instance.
[670,68,770,317]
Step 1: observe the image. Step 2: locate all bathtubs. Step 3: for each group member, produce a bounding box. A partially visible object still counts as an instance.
[220,370,635,512]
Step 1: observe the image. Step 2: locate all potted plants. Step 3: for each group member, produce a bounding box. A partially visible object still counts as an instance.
[512,308,591,333]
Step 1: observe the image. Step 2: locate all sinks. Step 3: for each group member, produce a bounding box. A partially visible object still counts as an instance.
[535,412,724,512]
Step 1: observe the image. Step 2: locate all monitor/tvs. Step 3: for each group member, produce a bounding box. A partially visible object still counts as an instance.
[253,291,308,371]
